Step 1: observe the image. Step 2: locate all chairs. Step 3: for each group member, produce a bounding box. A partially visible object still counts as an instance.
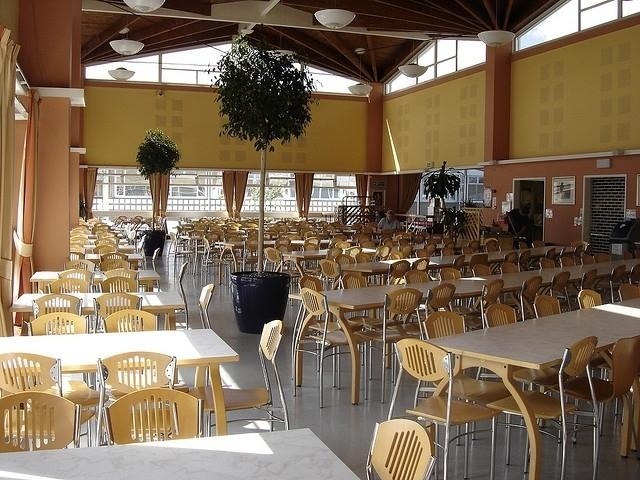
[0,216,640,480]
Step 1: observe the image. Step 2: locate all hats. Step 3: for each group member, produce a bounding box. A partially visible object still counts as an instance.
[384,210,394,216]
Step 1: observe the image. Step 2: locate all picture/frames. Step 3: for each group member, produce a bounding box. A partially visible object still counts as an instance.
[371,190,385,209]
[636,174,640,207]
[552,176,575,206]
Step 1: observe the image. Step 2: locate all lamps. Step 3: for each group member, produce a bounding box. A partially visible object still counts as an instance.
[107,57,136,82]
[110,28,145,57]
[314,0,356,29]
[477,0,516,47]
[347,48,373,97]
[398,40,428,77]
[119,0,167,14]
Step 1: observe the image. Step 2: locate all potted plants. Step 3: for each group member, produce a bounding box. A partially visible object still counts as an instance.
[135,126,183,256]
[203,36,320,335]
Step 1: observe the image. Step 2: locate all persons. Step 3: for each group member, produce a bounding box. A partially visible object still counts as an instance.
[504,205,529,236]
[378,210,400,231]
